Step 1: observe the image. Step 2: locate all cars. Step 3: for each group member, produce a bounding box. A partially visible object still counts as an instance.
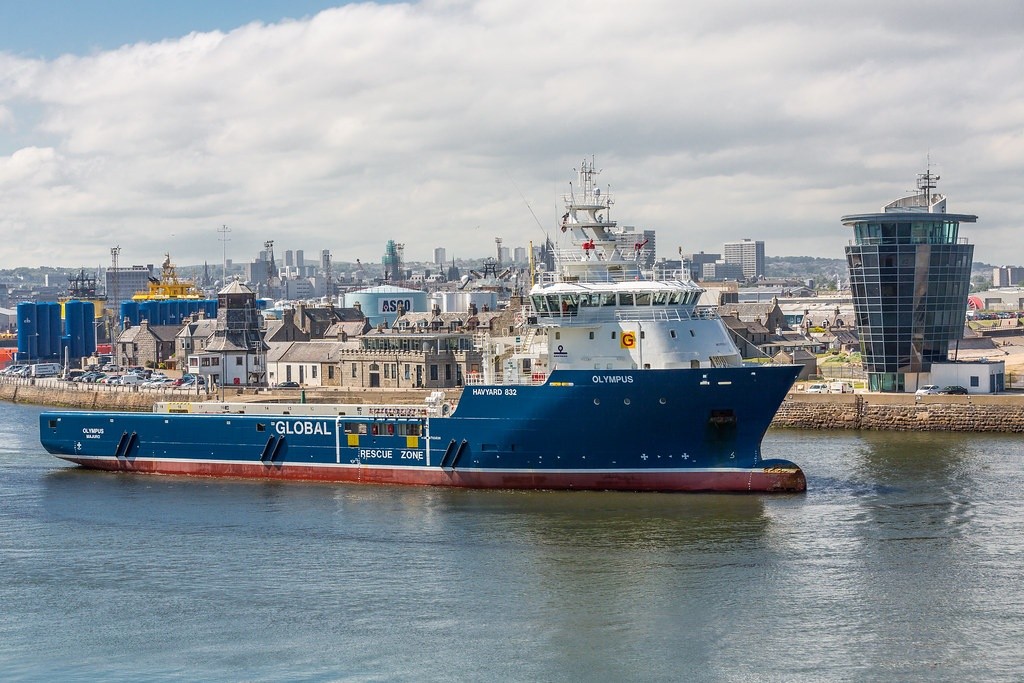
[808,383,830,394]
[181,373,196,382]
[830,382,854,394]
[141,378,162,387]
[73,372,97,382]
[277,382,300,387]
[180,379,205,386]
[105,376,121,384]
[172,378,184,386]
[968,311,1024,321]
[84,363,96,372]
[150,378,175,388]
[938,386,968,395]
[102,363,121,372]
[94,363,105,371]
[127,367,153,379]
[96,375,108,383]
[915,384,940,396]
[151,372,167,379]
[82,373,106,383]
[101,375,122,383]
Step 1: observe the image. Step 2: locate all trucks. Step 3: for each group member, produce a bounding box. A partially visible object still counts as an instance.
[32,363,64,378]
[120,375,147,385]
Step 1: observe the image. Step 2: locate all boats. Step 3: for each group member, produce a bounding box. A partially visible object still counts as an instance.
[39,151,808,494]
